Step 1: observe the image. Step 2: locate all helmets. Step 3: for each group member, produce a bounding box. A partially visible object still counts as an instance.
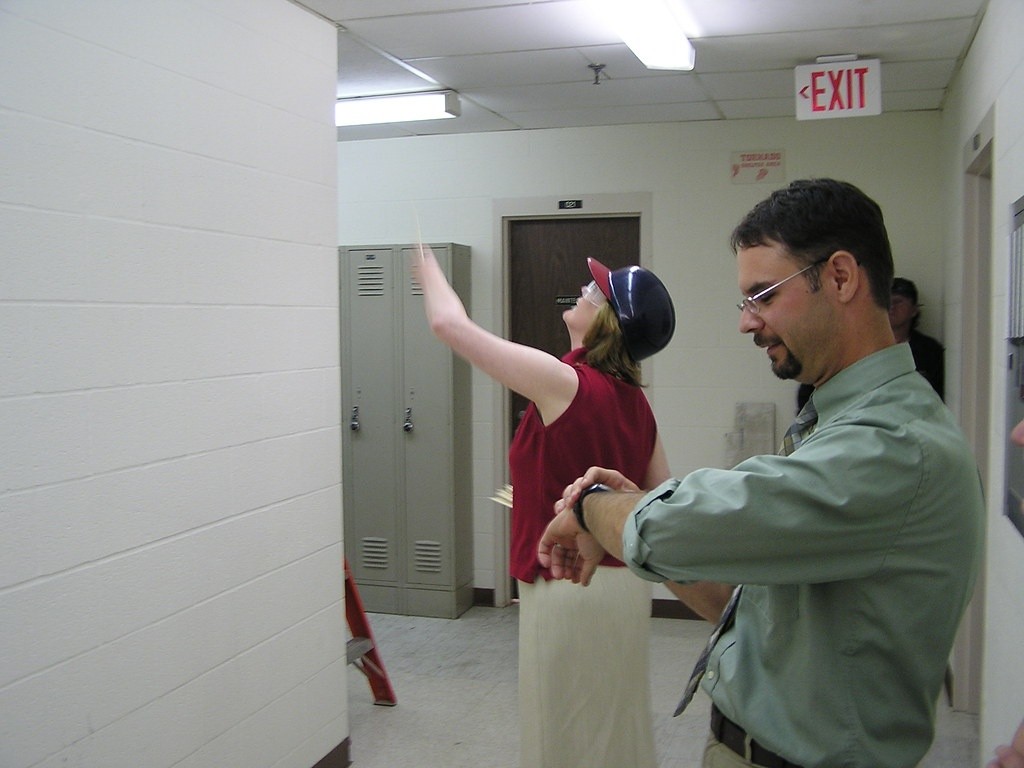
[587,256,676,363]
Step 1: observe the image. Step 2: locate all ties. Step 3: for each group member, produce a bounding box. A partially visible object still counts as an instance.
[673,396,817,717]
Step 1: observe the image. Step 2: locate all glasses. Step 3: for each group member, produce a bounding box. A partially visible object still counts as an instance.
[736,256,861,314]
[581,280,608,309]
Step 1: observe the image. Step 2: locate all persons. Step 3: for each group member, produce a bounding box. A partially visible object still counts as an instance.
[411,242,675,768]
[795,277,946,419]
[536,177,988,768]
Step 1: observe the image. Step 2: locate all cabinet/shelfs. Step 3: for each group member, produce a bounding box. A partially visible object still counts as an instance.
[337,242,474,620]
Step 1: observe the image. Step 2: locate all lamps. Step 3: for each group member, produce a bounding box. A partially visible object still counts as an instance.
[335,87,463,128]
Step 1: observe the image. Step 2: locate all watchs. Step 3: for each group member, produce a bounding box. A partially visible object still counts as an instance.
[572,483,616,535]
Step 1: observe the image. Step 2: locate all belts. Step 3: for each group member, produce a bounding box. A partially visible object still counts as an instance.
[710,701,805,768]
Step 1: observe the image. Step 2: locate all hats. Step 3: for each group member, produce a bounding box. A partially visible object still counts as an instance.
[892,277,919,302]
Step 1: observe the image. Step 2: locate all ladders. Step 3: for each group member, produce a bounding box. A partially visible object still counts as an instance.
[345,562,397,718]
